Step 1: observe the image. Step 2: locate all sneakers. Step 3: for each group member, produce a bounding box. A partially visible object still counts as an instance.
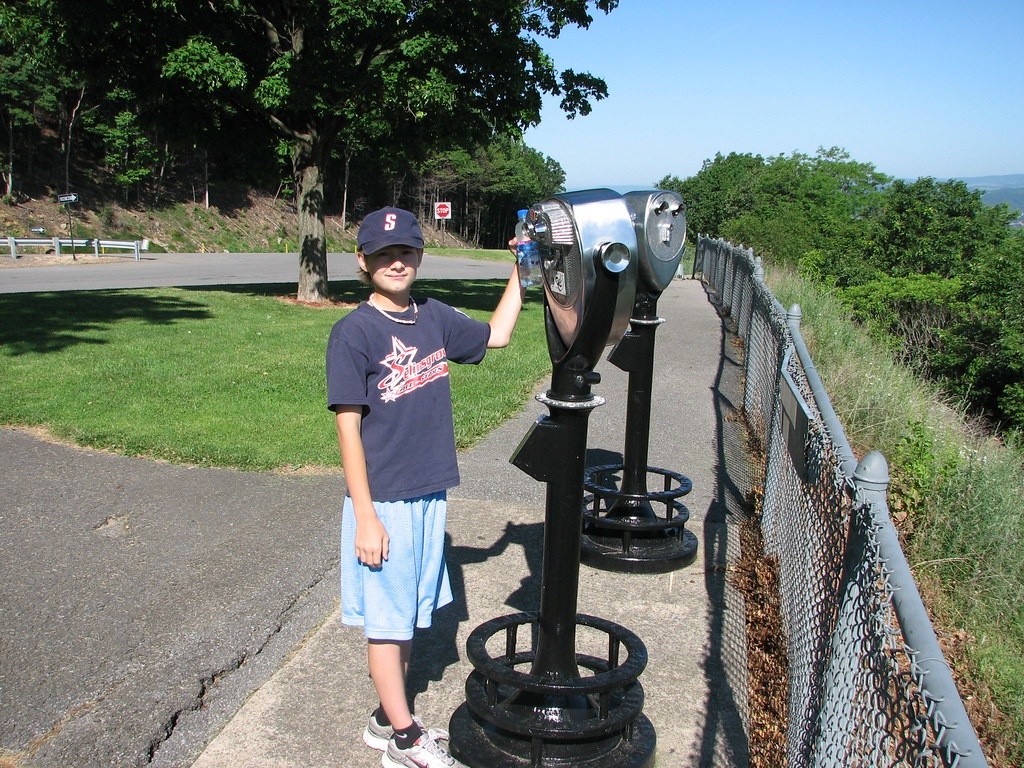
[382,728,470,768]
[362,706,431,752]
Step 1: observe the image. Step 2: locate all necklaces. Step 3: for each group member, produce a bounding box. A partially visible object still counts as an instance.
[369,293,418,324]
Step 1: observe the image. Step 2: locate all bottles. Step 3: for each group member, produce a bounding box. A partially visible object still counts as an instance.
[515,210,545,288]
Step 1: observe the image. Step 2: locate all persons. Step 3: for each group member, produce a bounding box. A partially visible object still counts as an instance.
[325,206,531,768]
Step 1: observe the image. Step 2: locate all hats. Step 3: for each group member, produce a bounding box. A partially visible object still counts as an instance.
[355,206,424,256]
